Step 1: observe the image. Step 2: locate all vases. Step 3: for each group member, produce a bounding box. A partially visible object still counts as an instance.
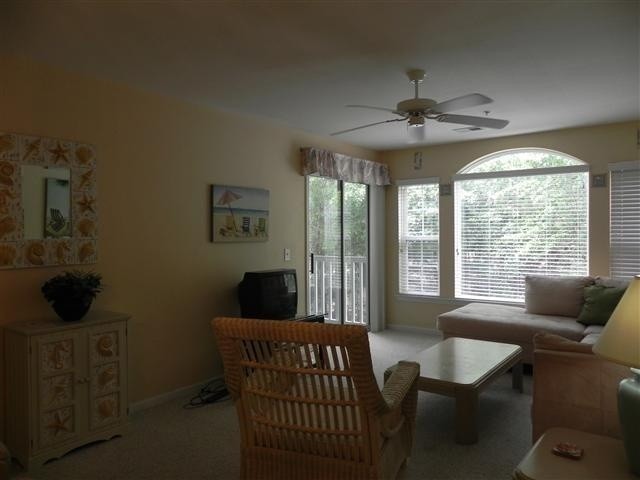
[616,367,640,480]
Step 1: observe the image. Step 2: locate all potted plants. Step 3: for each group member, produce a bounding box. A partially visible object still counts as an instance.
[40,263,103,321]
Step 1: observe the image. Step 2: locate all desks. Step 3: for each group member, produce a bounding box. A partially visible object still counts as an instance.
[513,425,640,480]
[290,314,326,369]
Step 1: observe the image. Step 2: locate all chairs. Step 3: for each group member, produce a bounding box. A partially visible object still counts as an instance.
[210,317,421,480]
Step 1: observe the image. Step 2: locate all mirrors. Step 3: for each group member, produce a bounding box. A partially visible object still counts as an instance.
[1,132,102,271]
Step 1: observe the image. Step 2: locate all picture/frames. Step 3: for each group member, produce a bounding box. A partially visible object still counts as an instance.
[211,183,270,243]
[43,176,74,238]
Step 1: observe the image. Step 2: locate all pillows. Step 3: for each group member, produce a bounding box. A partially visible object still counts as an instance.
[533,329,595,355]
[526,274,600,316]
[576,279,626,326]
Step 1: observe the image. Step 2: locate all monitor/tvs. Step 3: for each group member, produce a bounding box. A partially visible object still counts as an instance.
[237,268,298,324]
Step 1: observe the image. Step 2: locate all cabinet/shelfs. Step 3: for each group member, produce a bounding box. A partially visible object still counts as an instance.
[4,311,132,473]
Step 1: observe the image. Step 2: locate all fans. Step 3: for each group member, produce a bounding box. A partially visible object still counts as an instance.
[330,68,510,137]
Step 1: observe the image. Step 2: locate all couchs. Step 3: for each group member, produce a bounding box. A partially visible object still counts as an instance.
[436,275,640,443]
